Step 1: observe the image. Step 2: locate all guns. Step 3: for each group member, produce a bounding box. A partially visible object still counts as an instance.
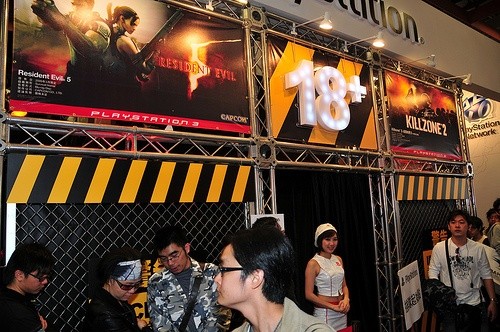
[128,8,184,75]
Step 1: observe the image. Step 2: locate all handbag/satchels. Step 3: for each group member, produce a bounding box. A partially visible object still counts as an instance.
[453,303,479,332]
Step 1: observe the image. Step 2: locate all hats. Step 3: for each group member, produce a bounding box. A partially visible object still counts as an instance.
[314,222,337,248]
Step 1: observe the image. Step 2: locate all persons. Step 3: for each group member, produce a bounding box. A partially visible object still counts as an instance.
[215,229,338,332]
[490,298,495,301]
[147,226,231,332]
[429,198,500,332]
[305,223,350,332]
[0,243,54,332]
[252,216,282,232]
[83,249,153,332]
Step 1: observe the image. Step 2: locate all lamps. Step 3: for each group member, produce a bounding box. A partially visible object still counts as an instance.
[437,74,472,86]
[291,11,333,35]
[344,32,384,52]
[397,54,435,71]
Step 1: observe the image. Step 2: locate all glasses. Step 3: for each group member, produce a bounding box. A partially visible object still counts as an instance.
[109,276,144,291]
[157,248,184,264]
[218,264,244,277]
[26,272,54,283]
